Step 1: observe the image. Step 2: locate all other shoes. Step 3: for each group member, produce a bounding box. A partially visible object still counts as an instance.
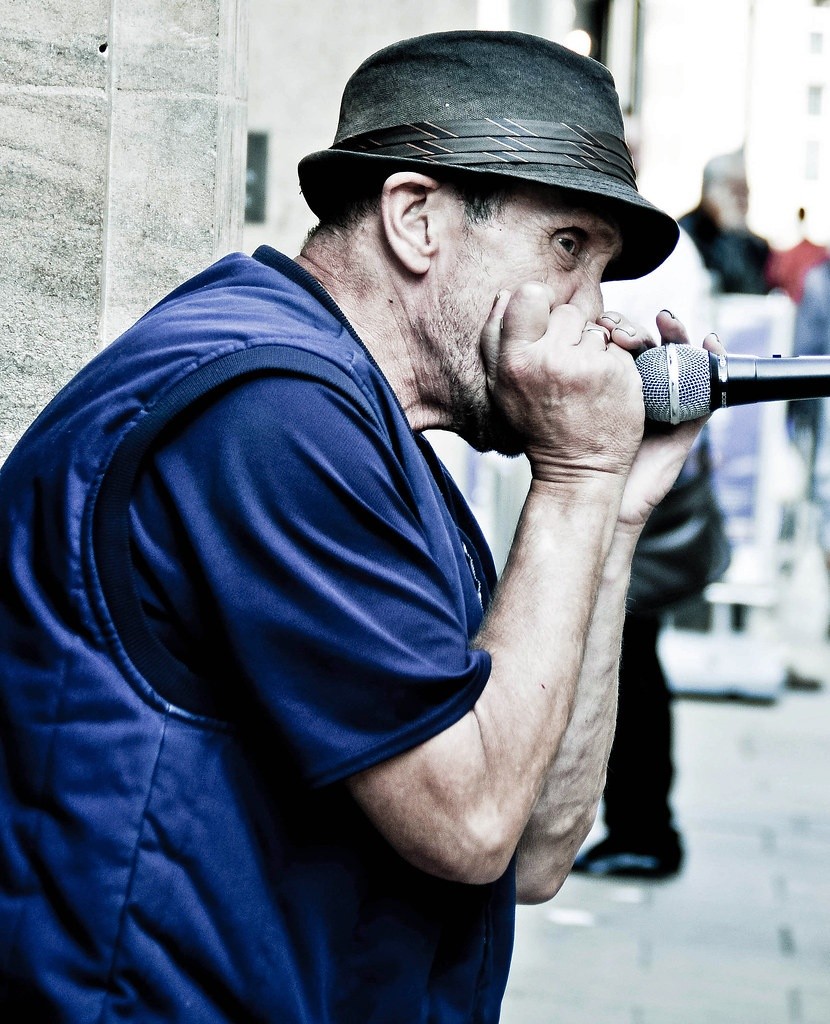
[570,839,685,878]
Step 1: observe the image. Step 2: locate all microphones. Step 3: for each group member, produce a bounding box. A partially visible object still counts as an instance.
[636,341,830,424]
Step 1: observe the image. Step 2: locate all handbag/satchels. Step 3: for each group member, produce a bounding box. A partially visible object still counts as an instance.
[626,475,718,605]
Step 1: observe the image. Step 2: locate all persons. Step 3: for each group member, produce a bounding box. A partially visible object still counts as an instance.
[0,28,729,1024]
[573,141,830,882]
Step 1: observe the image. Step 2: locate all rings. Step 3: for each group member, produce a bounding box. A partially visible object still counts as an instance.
[583,323,612,342]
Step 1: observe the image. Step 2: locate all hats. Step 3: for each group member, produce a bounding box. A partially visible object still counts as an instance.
[298,30,680,282]
